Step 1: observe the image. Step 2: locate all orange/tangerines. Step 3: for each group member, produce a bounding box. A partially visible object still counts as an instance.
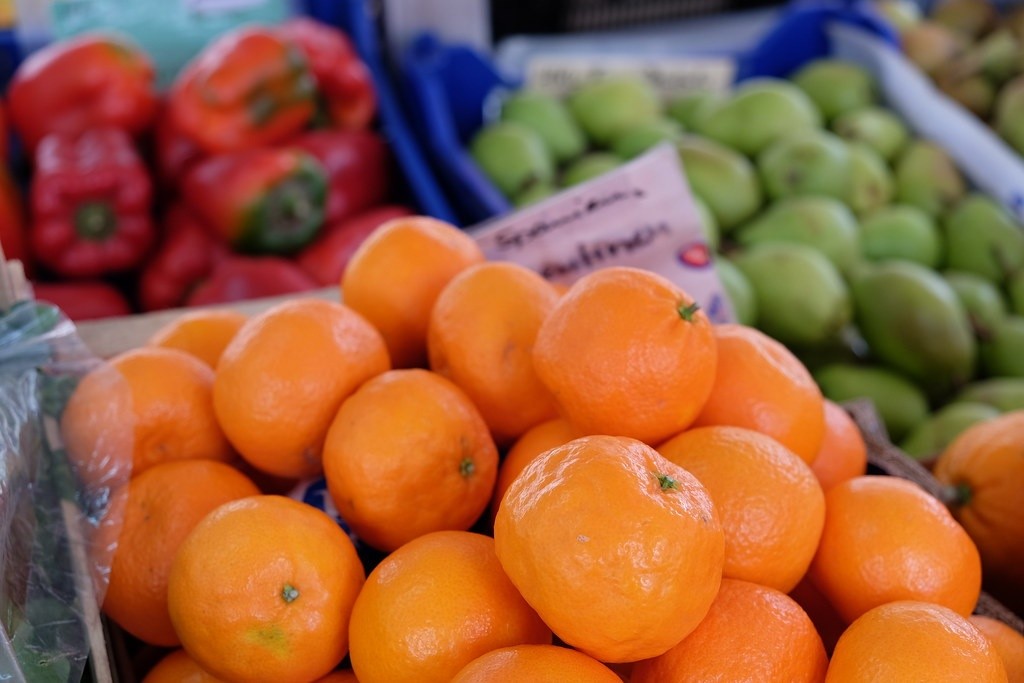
[63,215,1024,682]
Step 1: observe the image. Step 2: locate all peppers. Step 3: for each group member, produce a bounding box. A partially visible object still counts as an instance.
[0,18,409,325]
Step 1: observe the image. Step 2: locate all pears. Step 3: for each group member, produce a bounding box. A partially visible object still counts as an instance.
[467,55,1024,474]
